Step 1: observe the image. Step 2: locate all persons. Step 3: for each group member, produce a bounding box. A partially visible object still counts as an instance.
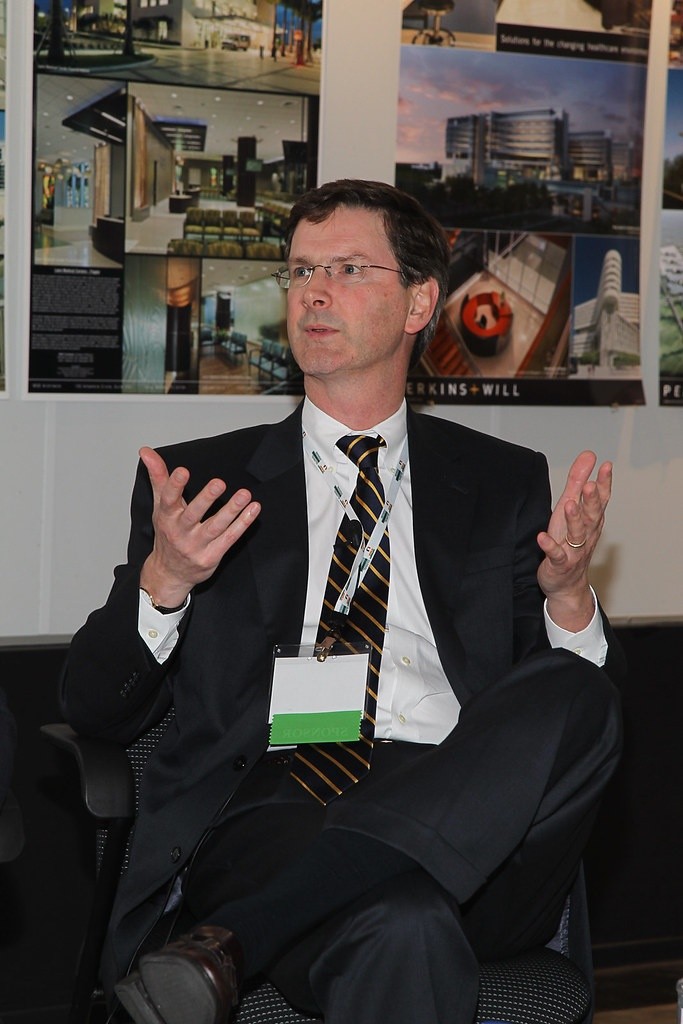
[67,179,625,1024]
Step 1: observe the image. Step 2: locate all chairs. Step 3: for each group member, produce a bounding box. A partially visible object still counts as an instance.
[40,708,588,1024]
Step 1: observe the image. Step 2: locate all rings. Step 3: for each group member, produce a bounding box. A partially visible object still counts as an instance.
[566,536,587,548]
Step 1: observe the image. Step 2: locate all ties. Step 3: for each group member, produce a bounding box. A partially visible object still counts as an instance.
[284,434,391,808]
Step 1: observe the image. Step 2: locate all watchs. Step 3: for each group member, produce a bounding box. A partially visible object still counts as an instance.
[140,587,187,614]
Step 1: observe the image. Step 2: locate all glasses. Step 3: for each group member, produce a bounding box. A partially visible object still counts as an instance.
[271,255,404,290]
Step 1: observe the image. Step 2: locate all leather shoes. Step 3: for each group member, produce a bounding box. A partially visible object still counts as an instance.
[113,924,243,1024]
